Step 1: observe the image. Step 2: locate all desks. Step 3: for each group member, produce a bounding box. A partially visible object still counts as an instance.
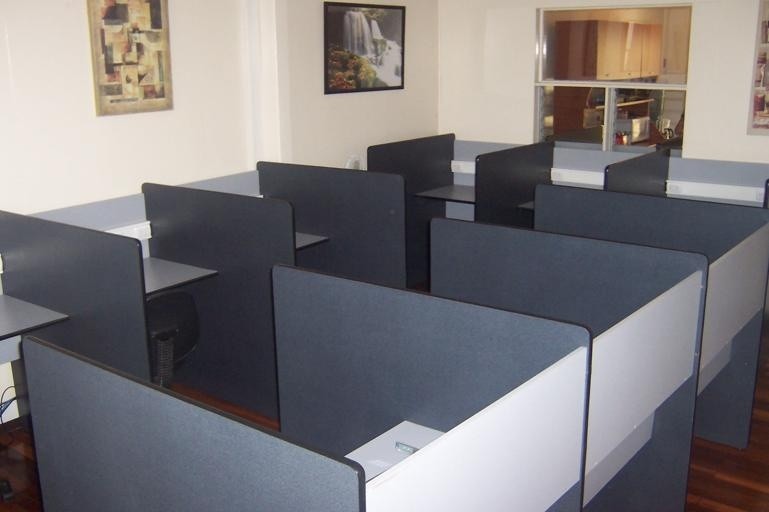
[1,135,768,511]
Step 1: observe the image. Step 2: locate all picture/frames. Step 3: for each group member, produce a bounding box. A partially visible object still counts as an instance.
[322,2,406,95]
[86,0,174,118]
